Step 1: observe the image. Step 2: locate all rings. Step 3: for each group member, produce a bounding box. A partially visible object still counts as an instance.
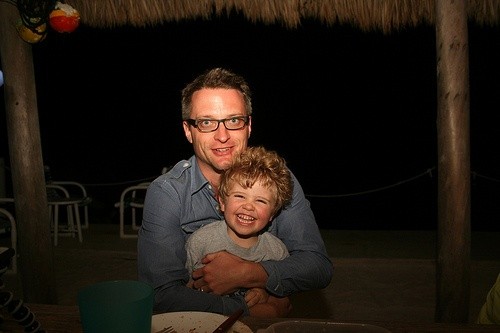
[200,286,205,293]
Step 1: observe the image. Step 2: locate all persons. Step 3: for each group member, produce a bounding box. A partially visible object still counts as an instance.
[136,68,334,318]
[185,145,295,309]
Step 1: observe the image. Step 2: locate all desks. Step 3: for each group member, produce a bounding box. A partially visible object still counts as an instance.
[0,301,500,333]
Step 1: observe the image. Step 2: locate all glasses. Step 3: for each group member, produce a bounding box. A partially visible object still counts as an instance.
[186,114,250,133]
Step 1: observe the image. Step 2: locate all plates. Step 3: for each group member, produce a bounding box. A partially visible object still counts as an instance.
[151,312,254,333]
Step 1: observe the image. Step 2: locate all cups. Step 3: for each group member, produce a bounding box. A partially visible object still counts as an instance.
[78,280,154,333]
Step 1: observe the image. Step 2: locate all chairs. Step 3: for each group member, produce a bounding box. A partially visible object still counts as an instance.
[114,167,172,239]
[45,181,92,247]
